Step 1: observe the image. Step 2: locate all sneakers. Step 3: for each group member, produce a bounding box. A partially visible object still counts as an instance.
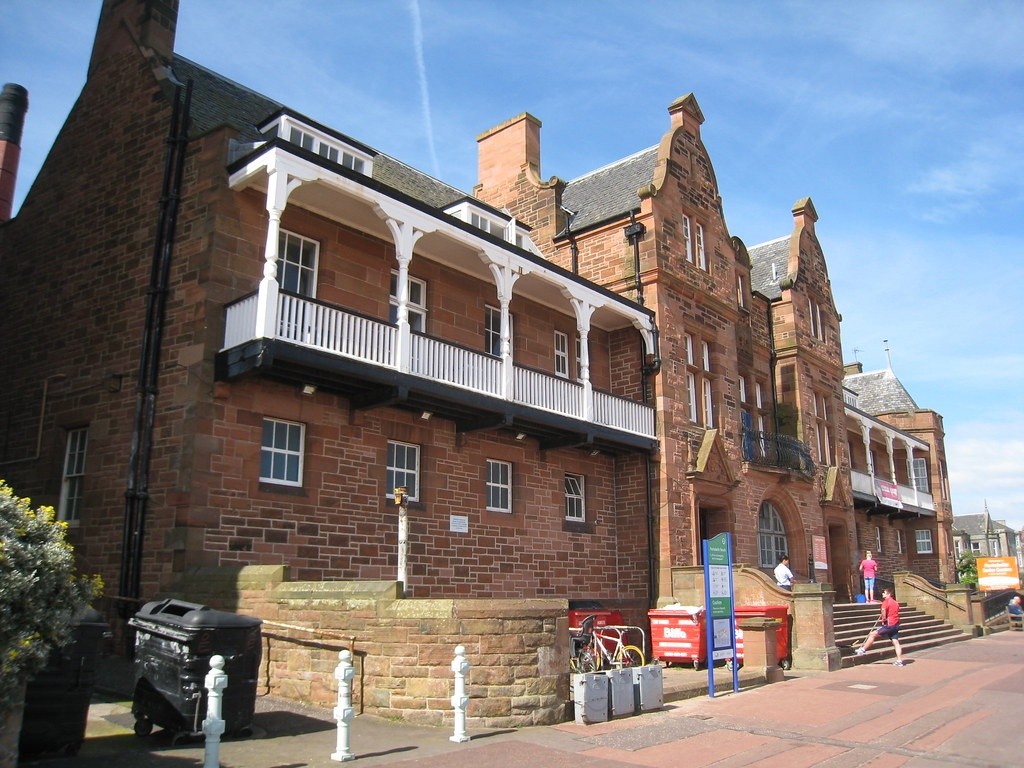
[870,600,878,603]
[866,599,870,603]
[892,660,904,666]
[854,647,865,653]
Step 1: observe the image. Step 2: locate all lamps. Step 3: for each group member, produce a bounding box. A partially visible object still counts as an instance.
[515,433,526,442]
[420,411,434,421]
[300,384,317,396]
[590,449,600,457]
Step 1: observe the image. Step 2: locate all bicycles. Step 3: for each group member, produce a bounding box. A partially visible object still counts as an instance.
[570,613,646,674]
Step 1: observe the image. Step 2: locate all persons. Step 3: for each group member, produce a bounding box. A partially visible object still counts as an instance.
[854,586,904,667]
[857,551,878,603]
[1008,596,1024,630]
[773,555,794,592]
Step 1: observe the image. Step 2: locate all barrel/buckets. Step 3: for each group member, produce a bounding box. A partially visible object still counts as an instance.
[856,594,866,603]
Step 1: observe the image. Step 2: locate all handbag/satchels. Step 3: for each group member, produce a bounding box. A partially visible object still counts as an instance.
[854,593,866,603]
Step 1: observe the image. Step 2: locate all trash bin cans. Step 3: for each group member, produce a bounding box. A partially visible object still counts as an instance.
[648,604,707,671]
[724,605,793,671]
[128,598,261,742]
[18,606,110,764]
[568,610,612,670]
